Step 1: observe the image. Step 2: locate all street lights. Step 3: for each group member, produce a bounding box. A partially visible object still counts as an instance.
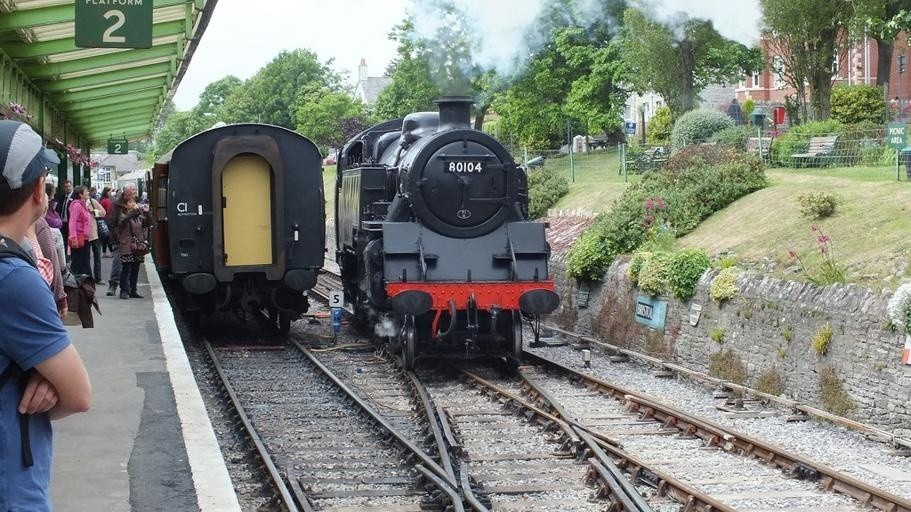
[638,105,647,146]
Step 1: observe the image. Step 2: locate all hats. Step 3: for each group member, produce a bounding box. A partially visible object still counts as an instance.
[0,118,61,191]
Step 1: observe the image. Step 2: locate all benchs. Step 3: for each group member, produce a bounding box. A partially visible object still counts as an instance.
[623,146,667,172]
[790,137,840,167]
[746,137,777,167]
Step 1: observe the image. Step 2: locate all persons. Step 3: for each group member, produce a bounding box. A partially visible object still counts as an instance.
[889,94,899,106]
[21,181,150,317]
[0,120,94,512]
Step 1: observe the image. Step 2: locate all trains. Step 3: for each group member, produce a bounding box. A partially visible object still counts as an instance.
[115,122,326,346]
[334,95,561,372]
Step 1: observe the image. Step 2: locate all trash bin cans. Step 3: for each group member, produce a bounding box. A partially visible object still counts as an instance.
[748,108,767,130]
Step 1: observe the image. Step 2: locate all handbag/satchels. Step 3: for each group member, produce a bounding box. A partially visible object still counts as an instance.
[68,231,85,248]
[96,217,113,244]
[130,240,150,257]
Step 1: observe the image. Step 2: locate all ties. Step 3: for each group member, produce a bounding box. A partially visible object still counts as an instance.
[62,196,68,219]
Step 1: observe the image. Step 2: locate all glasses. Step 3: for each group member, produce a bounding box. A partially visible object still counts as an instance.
[44,165,53,178]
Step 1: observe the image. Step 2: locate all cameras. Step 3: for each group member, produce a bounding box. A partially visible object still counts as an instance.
[139,203,149,210]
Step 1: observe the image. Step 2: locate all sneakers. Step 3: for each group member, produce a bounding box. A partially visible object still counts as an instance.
[106,284,116,296]
[120,289,129,299]
[130,292,144,298]
